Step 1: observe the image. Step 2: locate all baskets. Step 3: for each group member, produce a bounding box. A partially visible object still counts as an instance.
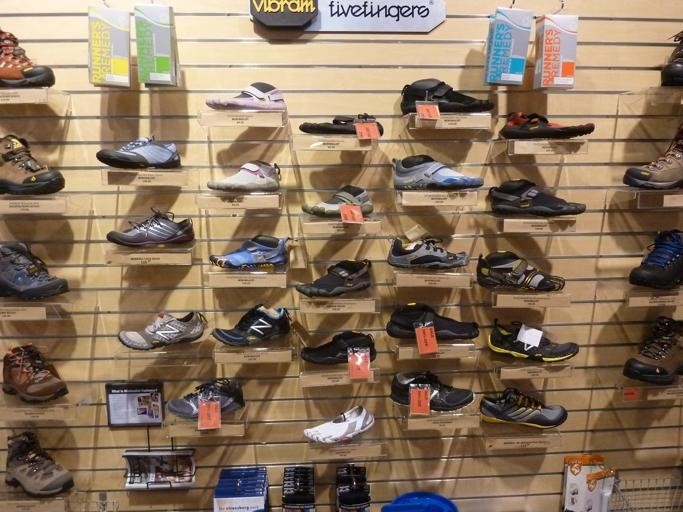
[607,478,683,512]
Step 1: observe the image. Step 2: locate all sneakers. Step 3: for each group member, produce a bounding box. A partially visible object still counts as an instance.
[386,303,479,340]
[207,160,282,192]
[106,206,194,247]
[387,235,470,269]
[5,432,74,499]
[629,229,683,290]
[480,387,568,429]
[167,377,244,418]
[488,319,580,362]
[298,113,384,137]
[206,81,287,110]
[0,135,65,195]
[622,316,682,385]
[297,258,372,298]
[490,178,586,216]
[118,311,204,350]
[212,303,292,347]
[476,251,565,292]
[0,30,55,88]
[623,140,682,190]
[0,242,68,300]
[96,135,180,169]
[301,331,377,365]
[301,185,374,218]
[499,111,595,140]
[661,31,683,86]
[2,343,69,404]
[391,155,484,191]
[400,79,494,114]
[389,370,475,413]
[304,405,374,445]
[209,236,288,271]
